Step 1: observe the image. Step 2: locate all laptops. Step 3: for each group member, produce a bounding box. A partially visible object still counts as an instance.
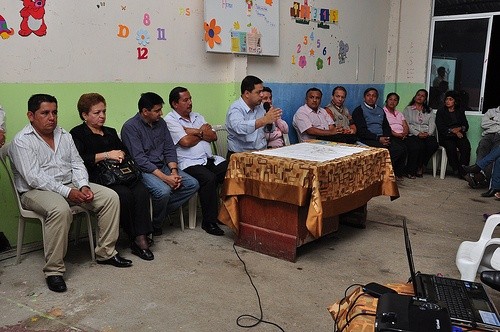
[402,216,500,332]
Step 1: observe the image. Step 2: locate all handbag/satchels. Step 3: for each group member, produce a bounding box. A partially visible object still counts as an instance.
[98,153,142,185]
[467,172,489,189]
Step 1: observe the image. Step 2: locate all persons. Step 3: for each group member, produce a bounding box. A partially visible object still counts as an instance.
[121,91,200,236]
[164,86,228,235]
[260,86,289,149]
[433,67,450,109]
[8,94,133,293]
[68,93,156,260]
[225,75,282,165]
[0,105,7,149]
[292,86,500,226]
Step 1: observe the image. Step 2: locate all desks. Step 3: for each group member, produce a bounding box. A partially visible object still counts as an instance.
[327,282,415,332]
[217,139,400,264]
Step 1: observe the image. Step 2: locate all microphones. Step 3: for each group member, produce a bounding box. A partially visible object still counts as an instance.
[264,102,272,127]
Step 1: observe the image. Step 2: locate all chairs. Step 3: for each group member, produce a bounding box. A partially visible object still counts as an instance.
[1,125,291,265]
[433,126,460,179]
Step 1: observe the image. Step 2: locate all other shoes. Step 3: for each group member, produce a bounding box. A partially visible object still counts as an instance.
[405,172,416,179]
[458,169,469,182]
[482,211,500,220]
[415,168,423,178]
[152,226,163,235]
[494,192,500,200]
[395,174,403,181]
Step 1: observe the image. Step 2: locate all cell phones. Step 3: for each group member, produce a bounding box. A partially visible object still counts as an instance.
[362,282,396,297]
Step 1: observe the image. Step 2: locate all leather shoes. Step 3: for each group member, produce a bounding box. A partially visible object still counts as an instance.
[146,236,154,247]
[214,219,226,225]
[132,241,154,261]
[202,221,224,234]
[480,188,500,197]
[462,165,481,173]
[97,254,132,267]
[46,275,67,292]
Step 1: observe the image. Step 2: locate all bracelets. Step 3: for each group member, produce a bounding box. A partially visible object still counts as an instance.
[104,152,109,160]
[170,168,178,174]
[200,130,203,138]
